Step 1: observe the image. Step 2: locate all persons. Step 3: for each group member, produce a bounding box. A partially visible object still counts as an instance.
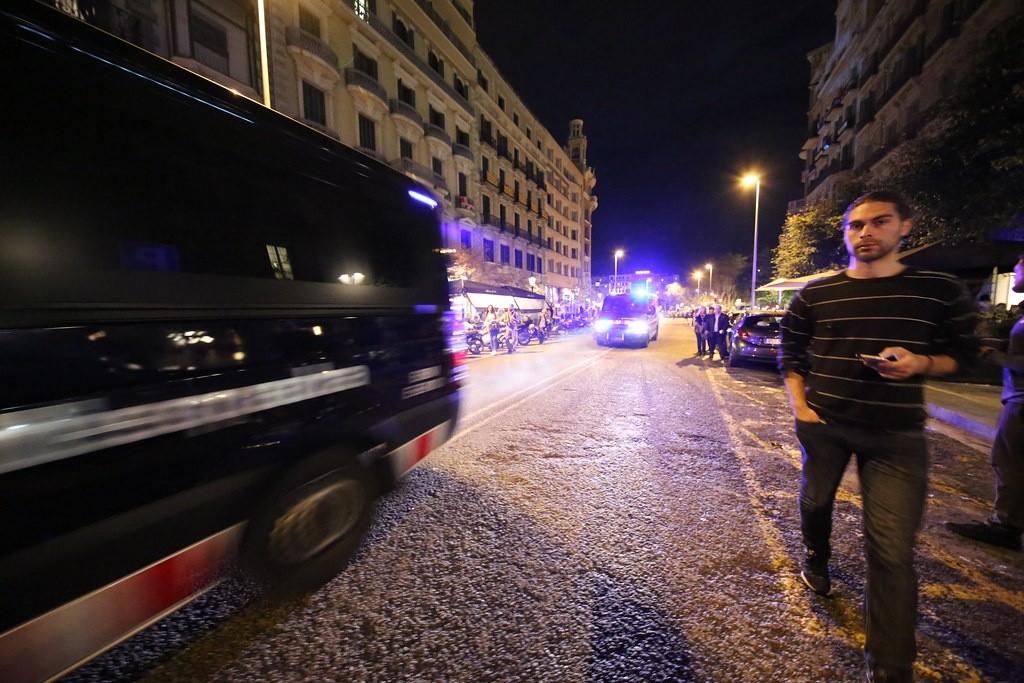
[944,255,1024,551]
[695,305,731,360]
[483,303,600,356]
[778,192,978,683]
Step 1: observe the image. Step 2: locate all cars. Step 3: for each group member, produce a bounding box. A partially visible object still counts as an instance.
[727,310,752,353]
[728,310,785,367]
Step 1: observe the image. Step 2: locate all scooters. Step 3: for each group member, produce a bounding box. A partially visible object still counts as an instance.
[466,311,593,355]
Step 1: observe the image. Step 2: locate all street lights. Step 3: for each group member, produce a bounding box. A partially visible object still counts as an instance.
[742,175,760,310]
[528,272,536,293]
[646,278,652,290]
[706,264,712,290]
[695,272,702,304]
[615,250,623,291]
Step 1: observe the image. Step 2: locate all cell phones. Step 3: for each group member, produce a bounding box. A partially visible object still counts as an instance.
[855,353,889,363]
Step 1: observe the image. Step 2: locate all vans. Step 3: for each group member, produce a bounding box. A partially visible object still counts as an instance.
[595,294,659,348]
[0,0,460,683]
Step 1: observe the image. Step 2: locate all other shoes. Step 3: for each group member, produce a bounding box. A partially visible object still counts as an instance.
[709,354,713,358]
[702,351,705,355]
[698,351,701,356]
[492,350,496,356]
[944,521,1021,551]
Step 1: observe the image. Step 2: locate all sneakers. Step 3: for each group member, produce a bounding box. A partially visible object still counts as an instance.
[801,552,833,595]
